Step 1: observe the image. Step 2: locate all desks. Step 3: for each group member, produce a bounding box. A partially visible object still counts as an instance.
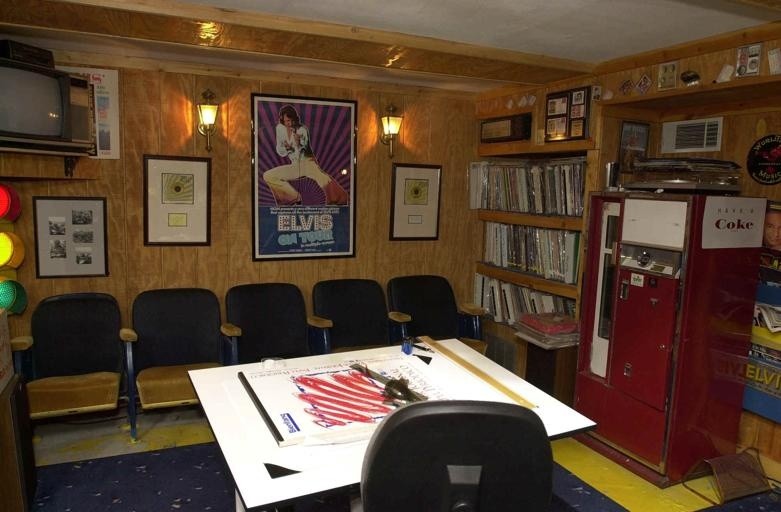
[187,339,597,512]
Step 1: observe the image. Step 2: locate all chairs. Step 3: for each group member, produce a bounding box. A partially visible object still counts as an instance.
[130,288,235,433]
[313,279,405,353]
[389,272,480,353]
[347,399,555,512]
[12,292,134,444]
[225,282,328,367]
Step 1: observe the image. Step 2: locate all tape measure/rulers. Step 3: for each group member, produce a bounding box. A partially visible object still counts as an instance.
[350,364,428,400]
[419,335,537,409]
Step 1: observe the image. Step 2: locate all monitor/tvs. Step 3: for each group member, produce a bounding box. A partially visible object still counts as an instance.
[0,58,72,142]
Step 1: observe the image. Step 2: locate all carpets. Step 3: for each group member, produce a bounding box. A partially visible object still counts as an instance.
[695,488,781,512]
[27,440,630,512]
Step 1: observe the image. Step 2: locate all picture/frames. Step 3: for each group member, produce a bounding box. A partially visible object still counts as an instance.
[544,86,589,142]
[251,93,357,261]
[33,196,108,279]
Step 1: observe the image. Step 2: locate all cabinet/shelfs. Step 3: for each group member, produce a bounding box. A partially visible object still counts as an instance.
[475,139,598,406]
[143,154,211,246]
[572,191,768,489]
[389,162,442,241]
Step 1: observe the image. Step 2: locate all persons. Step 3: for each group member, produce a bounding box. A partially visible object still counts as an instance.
[763,211,781,251]
[263,104,349,207]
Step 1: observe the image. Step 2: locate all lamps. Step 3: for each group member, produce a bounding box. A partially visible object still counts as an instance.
[196,89,219,152]
[379,103,403,159]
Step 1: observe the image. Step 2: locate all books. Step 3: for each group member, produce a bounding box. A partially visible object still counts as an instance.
[466,153,581,350]
